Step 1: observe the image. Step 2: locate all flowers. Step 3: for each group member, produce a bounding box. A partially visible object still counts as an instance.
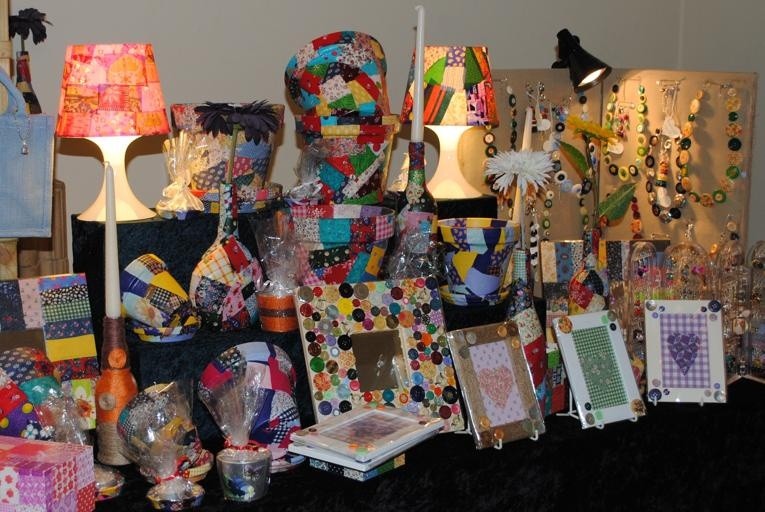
[484,149,557,251]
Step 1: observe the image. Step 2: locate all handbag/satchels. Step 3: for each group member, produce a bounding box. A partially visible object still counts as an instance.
[0,68,58,238]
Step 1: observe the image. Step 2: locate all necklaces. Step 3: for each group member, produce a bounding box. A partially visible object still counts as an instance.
[475,80,743,245]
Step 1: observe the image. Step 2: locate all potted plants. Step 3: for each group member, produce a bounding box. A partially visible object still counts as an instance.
[8,8,53,114]
[190,99,278,333]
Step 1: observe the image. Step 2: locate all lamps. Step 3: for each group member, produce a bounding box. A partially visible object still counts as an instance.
[551,28,612,93]
[55,40,170,464]
[398,45,500,200]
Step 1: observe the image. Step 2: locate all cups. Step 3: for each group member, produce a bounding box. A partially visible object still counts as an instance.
[216,447,270,503]
[258,292,298,332]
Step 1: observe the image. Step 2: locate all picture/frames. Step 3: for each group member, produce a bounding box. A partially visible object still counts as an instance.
[646,298,726,405]
[288,401,443,472]
[294,274,465,435]
[553,309,647,429]
[446,320,546,449]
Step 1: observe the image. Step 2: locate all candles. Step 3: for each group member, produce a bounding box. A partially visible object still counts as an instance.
[102,161,121,320]
[410,3,427,142]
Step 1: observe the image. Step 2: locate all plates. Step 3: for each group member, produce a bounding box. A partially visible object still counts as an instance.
[133,325,199,344]
[182,182,285,215]
[439,286,512,307]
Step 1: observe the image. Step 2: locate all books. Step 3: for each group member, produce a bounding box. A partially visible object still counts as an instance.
[0,237,100,431]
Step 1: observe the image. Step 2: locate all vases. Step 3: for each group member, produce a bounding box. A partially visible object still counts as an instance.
[161,102,286,213]
[118,383,199,457]
[506,251,552,420]
[435,217,521,305]
[272,205,396,286]
[119,252,189,337]
[196,339,302,461]
